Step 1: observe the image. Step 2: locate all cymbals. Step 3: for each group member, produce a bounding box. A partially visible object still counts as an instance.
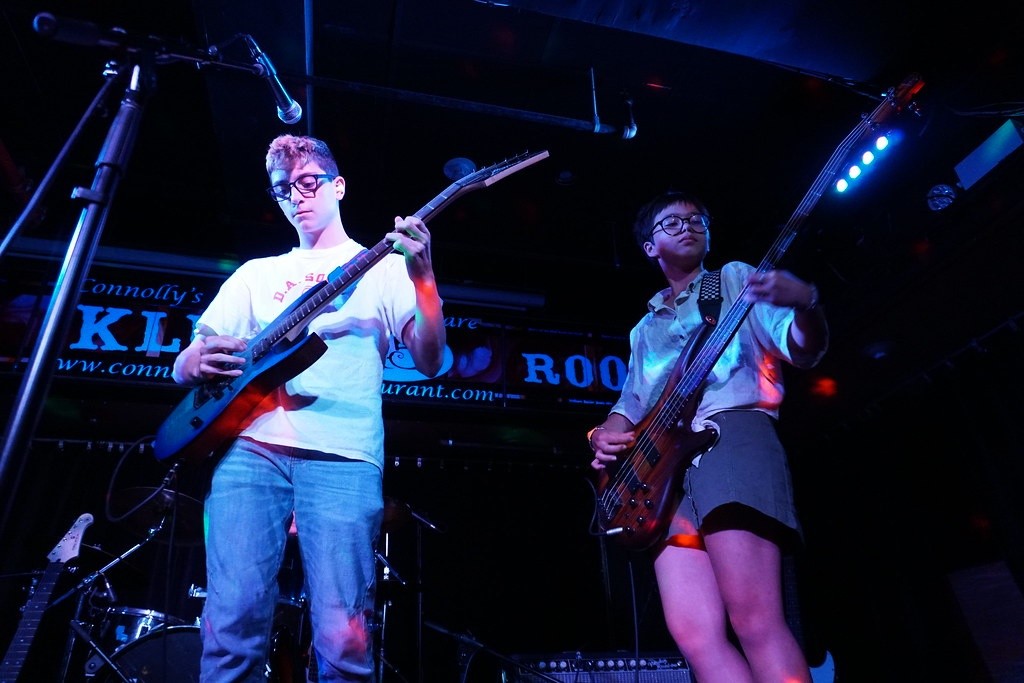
[130,485,205,506]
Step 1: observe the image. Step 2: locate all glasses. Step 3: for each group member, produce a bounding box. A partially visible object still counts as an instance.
[646,214,711,239]
[266,174,338,201]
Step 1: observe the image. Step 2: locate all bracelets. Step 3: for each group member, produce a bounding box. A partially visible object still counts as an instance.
[793,284,819,318]
[587,425,608,452]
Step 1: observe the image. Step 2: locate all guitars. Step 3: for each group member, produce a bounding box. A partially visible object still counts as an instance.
[0,513,95,682]
[152,145,547,463]
[593,72,929,545]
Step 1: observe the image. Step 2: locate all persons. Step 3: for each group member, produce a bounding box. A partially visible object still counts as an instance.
[172,135,448,683]
[591,189,831,683]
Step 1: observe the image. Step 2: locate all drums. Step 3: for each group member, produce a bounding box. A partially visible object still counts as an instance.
[98,604,188,650]
[87,625,209,683]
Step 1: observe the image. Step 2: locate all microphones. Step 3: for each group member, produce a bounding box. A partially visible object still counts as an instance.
[245,36,303,123]
